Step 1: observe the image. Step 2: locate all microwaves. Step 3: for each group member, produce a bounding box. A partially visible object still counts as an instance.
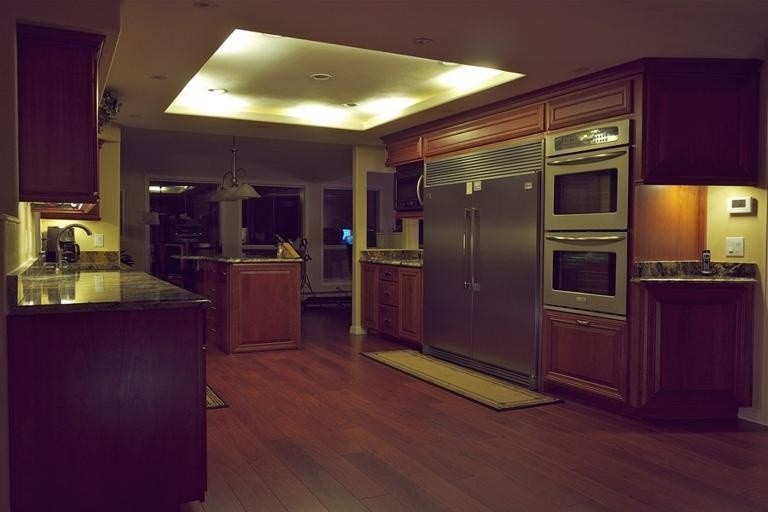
[393,159,423,211]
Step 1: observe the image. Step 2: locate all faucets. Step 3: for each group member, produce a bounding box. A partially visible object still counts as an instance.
[56,224,92,271]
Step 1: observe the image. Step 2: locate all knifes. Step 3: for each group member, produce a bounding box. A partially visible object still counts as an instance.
[274,232,288,243]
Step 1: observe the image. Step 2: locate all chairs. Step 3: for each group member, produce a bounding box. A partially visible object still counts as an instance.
[159,219,191,271]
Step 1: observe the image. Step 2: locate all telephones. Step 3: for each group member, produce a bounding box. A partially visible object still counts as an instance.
[701,250,713,275]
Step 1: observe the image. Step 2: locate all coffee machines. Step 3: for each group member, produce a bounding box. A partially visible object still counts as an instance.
[46,227,80,263]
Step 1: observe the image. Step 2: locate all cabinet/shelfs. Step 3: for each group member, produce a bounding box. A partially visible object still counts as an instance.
[40,140,102,221]
[377,264,400,336]
[199,260,301,349]
[542,308,627,406]
[400,266,423,349]
[7,305,207,511]
[16,24,107,202]
[360,263,377,332]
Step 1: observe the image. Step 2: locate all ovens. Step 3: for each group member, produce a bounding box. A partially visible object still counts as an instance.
[544,118,630,321]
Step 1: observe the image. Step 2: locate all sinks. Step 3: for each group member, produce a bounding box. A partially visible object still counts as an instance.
[64,264,136,272]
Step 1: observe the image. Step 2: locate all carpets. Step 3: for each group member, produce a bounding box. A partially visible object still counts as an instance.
[206,383,228,409]
[360,349,566,411]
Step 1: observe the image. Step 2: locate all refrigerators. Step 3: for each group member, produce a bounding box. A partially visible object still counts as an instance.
[421,138,542,393]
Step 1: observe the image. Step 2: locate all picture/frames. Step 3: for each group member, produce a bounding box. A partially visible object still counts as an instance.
[121,184,128,241]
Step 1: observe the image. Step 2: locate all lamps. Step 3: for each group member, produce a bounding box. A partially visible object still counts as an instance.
[208,134,262,203]
